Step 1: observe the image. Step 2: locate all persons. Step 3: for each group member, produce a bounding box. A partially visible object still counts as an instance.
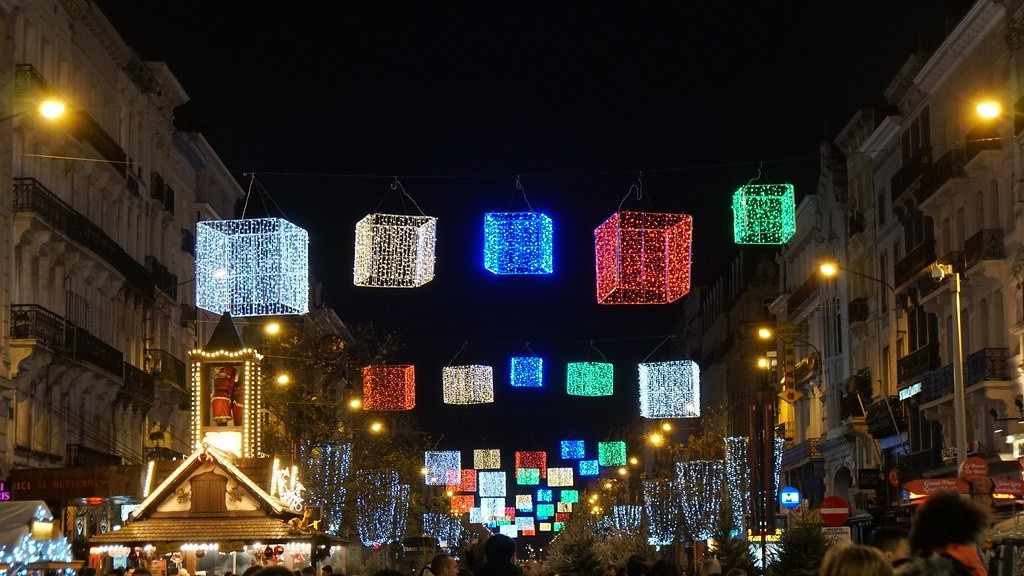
[419,534,772,576]
[77,565,337,576]
[816,490,988,576]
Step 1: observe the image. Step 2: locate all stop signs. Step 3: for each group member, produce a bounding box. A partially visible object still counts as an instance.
[820,496,850,527]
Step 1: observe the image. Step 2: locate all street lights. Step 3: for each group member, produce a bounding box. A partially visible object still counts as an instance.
[931,261,966,477]
[531,548,542,562]
